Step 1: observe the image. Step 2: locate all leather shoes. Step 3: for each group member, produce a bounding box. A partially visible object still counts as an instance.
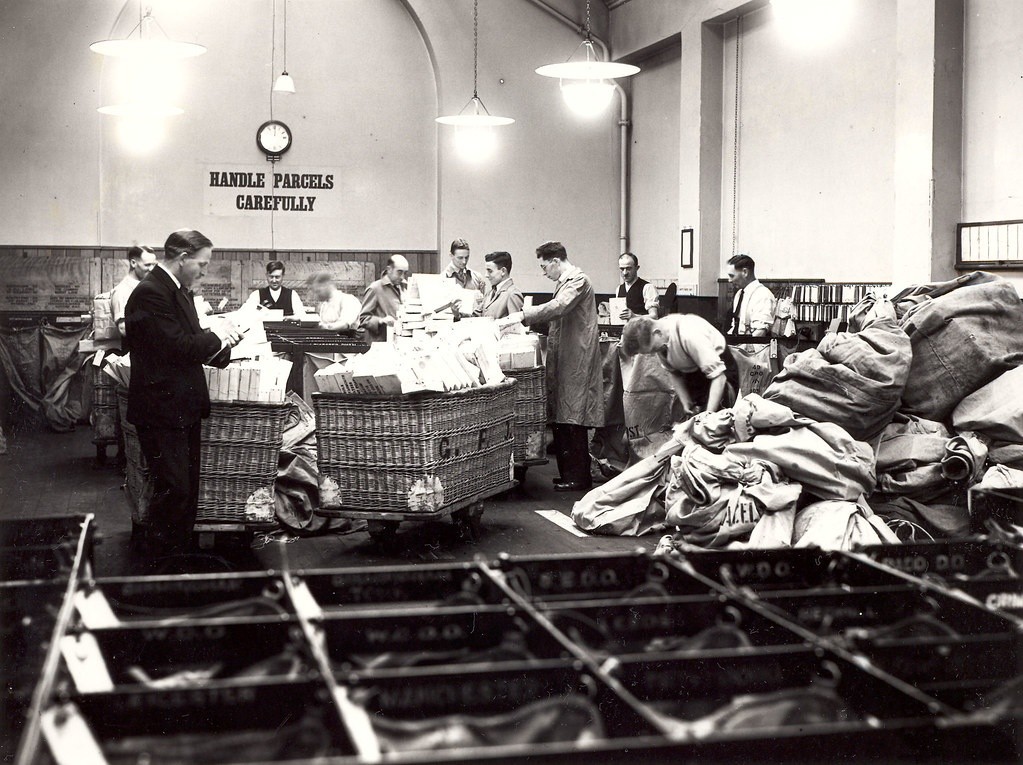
[554,482,586,490]
[553,477,564,484]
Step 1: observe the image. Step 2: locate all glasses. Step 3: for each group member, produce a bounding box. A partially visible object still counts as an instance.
[540,260,553,270]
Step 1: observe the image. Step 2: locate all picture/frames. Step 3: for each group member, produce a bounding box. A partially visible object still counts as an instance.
[679,227,695,269]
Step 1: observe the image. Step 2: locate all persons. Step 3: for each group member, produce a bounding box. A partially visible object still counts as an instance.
[359,255,409,345]
[623,314,740,419]
[112,246,157,351]
[441,238,492,296]
[495,241,605,491]
[238,261,306,321]
[307,273,362,329]
[616,253,659,320]
[125,229,245,554]
[450,251,524,322]
[726,255,777,337]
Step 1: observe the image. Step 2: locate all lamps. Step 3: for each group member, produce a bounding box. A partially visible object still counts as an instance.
[535,0,643,123]
[431,0,518,152]
[272,0,298,95]
[82,0,210,100]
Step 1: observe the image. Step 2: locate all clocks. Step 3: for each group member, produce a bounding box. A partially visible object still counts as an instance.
[256,119,292,154]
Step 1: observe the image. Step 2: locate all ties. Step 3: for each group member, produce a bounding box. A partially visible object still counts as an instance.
[732,290,744,334]
[459,269,464,280]
[491,287,497,300]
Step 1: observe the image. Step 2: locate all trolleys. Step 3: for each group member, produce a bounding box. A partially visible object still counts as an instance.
[80,329,125,473]
[496,365,553,484]
[111,382,295,552]
[309,376,520,545]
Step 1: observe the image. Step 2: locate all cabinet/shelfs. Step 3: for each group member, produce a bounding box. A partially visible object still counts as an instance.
[789,283,893,325]
